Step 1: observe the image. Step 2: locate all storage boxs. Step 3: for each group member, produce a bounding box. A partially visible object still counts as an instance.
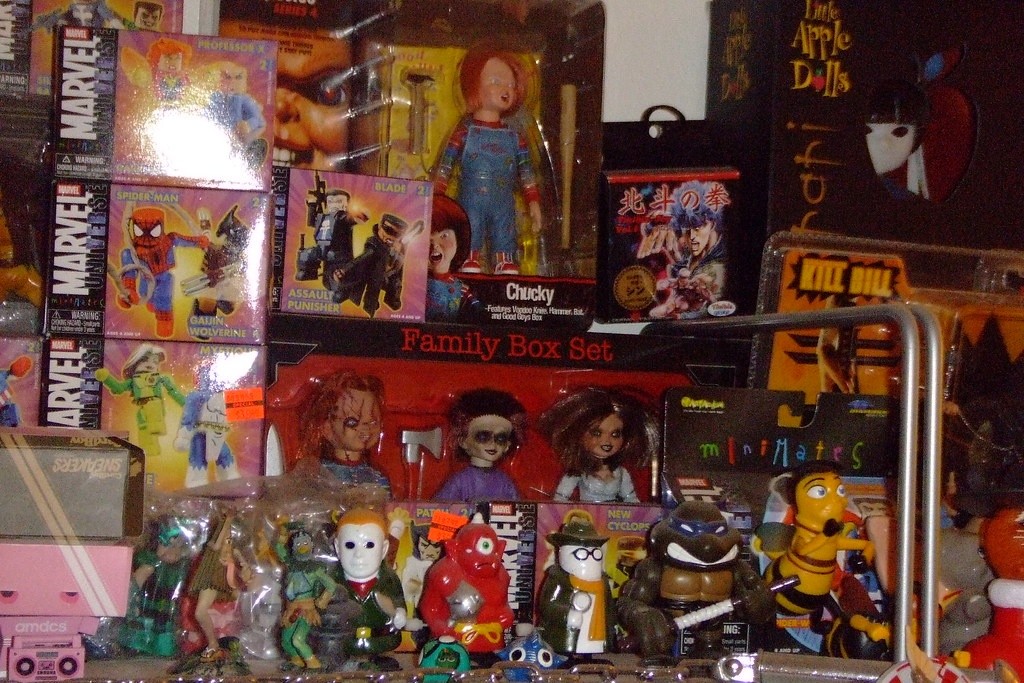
[0,0,1024,683]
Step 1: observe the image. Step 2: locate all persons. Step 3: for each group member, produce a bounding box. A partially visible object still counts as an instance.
[534,510,616,671]
[95,343,185,460]
[171,360,242,490]
[333,214,407,316]
[288,366,393,506]
[274,519,337,678]
[294,189,368,292]
[78,499,271,683]
[425,196,483,324]
[421,43,543,275]
[336,506,404,657]
[92,0,163,32]
[427,386,529,504]
[536,386,663,506]
